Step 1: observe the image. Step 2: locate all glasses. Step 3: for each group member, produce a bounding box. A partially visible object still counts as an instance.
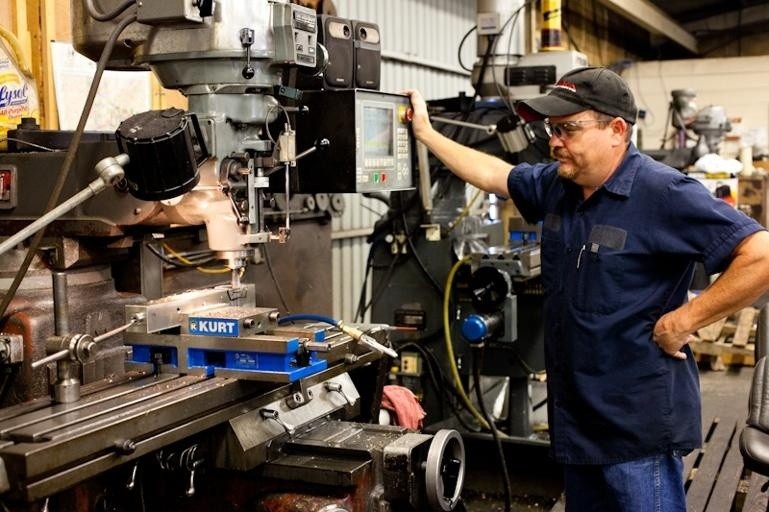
[542,118,605,140]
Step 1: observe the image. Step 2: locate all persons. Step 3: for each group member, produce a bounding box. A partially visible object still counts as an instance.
[400,66,768,512]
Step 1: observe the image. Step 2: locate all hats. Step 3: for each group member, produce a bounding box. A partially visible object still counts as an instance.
[515,66,637,126]
